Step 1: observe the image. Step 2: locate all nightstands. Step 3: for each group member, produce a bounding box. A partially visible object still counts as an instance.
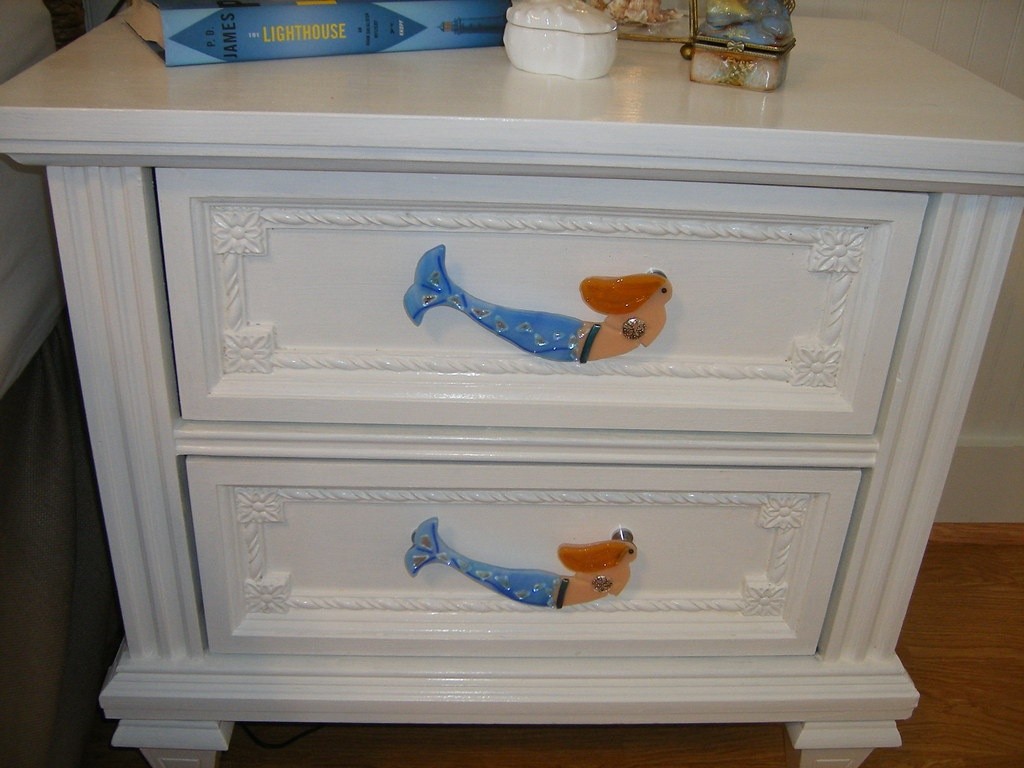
[0,0,1024,768]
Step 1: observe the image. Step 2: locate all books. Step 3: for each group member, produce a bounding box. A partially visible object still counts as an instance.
[118,0,513,68]
